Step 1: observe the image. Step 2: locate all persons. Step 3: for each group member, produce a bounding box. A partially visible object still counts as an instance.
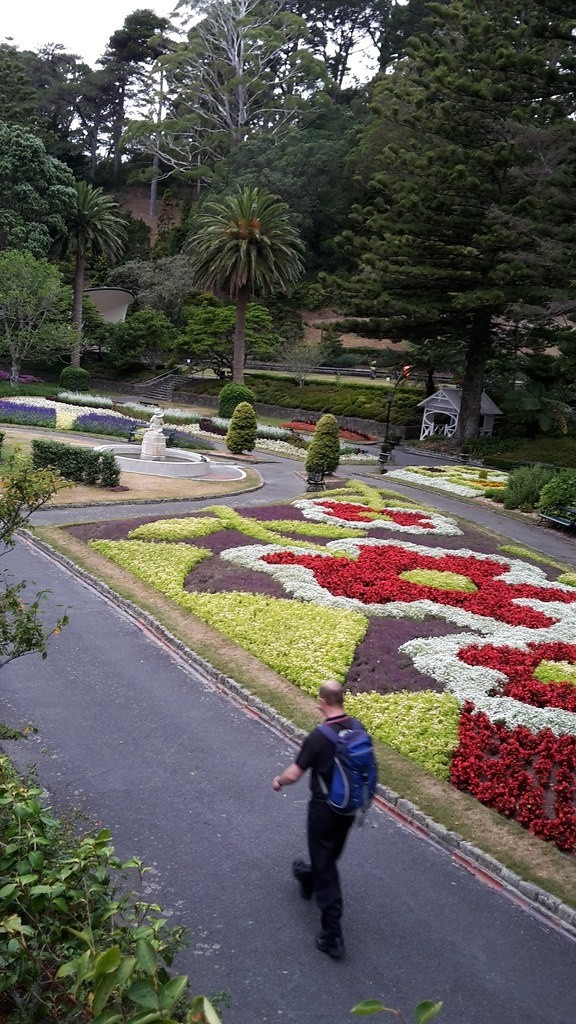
[272,680,372,960]
[149,408,164,433]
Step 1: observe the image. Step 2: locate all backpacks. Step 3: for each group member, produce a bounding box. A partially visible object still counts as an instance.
[313,718,378,816]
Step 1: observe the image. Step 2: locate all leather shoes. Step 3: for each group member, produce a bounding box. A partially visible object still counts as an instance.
[316,931,344,956]
[293,859,312,900]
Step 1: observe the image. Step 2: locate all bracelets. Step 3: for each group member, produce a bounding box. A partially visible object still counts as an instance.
[277,776,284,787]
